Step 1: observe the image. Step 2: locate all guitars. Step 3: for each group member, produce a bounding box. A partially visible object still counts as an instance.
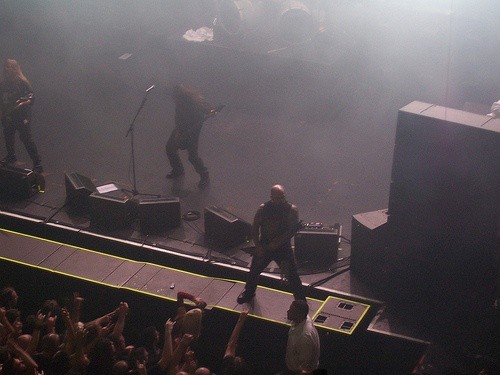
[169,102,227,150]
[239,219,307,258]
[0,93,36,129]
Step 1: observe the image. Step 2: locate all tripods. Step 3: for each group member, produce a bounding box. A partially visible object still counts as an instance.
[122,91,161,201]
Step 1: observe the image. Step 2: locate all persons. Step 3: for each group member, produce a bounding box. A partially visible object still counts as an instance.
[0,58,43,173]
[237,185,307,304]
[0,288,328,375]
[165,84,210,187]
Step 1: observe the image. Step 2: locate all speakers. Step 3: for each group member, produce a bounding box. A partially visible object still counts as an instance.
[65,173,99,215]
[293,228,339,263]
[204,206,253,247]
[350,209,388,288]
[0,162,45,198]
[139,197,181,226]
[89,188,134,219]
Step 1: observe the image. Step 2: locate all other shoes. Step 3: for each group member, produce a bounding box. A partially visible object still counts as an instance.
[33,162,42,172]
[198,177,210,187]
[237,289,256,304]
[166,171,185,179]
[0,154,16,164]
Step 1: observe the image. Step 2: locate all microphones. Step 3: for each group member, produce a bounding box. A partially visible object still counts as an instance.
[146,85,155,92]
